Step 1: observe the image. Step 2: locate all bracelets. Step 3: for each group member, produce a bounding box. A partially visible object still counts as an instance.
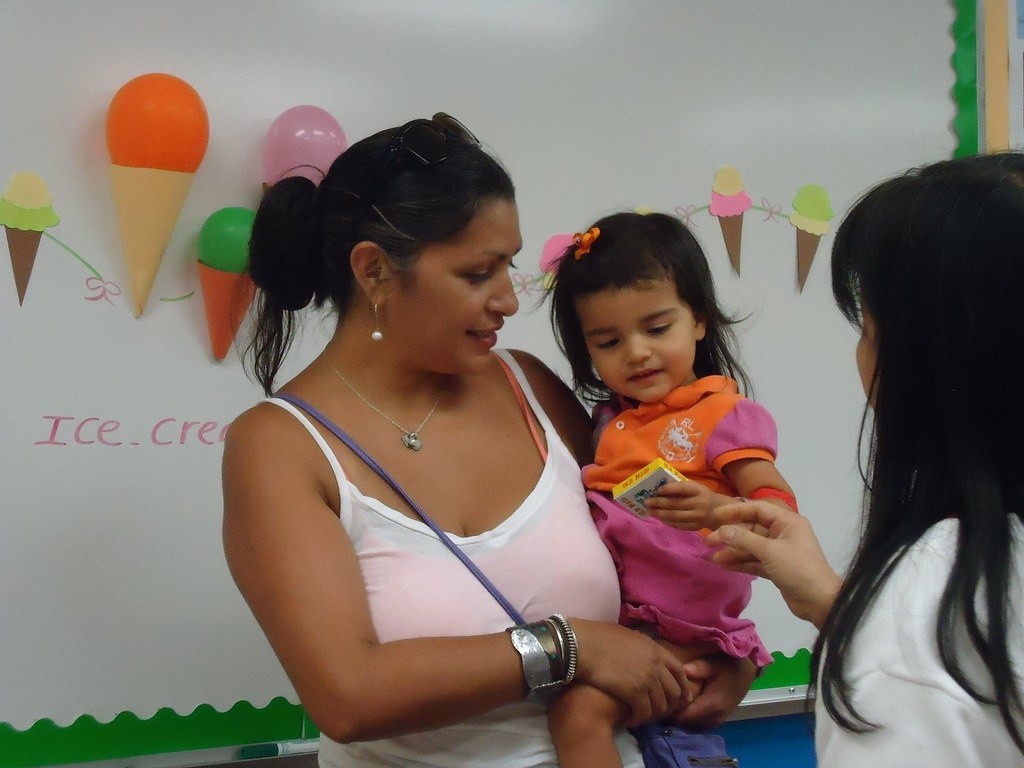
[506,615,579,700]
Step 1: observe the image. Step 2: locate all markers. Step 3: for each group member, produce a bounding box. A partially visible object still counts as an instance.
[234,736,318,762]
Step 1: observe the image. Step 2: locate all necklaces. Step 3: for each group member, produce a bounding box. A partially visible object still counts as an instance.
[322,342,445,449]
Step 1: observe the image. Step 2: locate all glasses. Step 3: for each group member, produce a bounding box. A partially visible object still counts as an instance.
[359,112,482,202]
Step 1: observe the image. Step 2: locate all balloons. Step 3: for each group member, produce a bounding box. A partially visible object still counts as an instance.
[106,72,211,172]
[262,106,346,187]
[197,207,257,274]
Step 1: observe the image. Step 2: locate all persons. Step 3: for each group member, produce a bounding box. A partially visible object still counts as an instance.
[222,113,763,768]
[705,152,1024,768]
[548,212,800,768]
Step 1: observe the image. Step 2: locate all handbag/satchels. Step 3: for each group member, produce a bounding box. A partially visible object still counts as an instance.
[627,726,738,768]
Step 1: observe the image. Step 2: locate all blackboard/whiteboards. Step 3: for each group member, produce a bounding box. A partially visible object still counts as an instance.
[0,0,985,768]
